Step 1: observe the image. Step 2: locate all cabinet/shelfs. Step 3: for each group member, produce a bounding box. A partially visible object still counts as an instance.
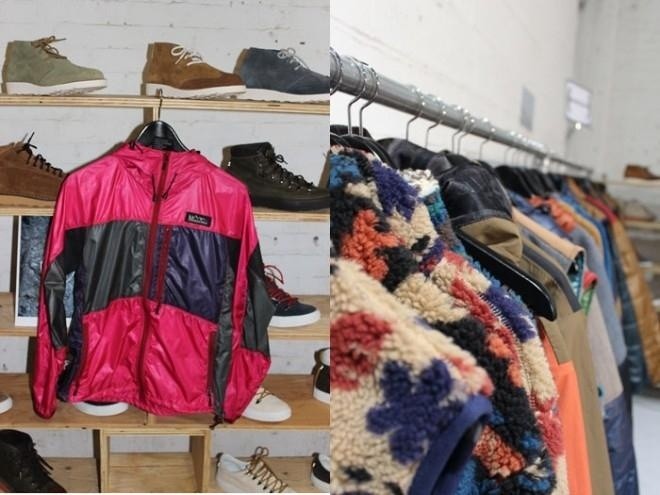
[0,93,331,494]
[600,175,660,313]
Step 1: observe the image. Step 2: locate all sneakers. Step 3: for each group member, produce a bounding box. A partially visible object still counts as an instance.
[312,452,331,492]
[260,265,321,328]
[3,36,108,95]
[623,199,654,221]
[311,348,330,405]
[0,429,66,493]
[0,131,67,208]
[625,166,660,180]
[233,47,331,103]
[143,42,246,98]
[215,446,295,493]
[226,144,332,210]
[244,388,292,421]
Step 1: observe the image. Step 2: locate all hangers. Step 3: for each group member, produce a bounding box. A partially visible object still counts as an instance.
[136,98,187,152]
